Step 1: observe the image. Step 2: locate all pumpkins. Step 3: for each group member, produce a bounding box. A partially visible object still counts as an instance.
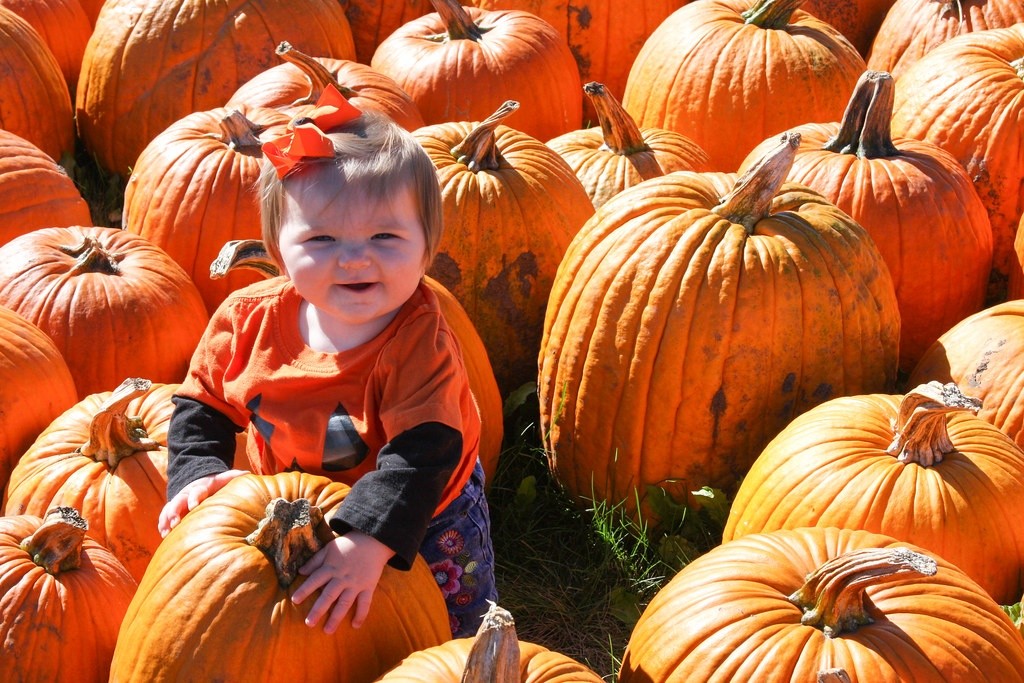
[0,0,1024,683]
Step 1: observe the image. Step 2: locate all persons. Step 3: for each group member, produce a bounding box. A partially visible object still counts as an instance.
[158,84,498,641]
